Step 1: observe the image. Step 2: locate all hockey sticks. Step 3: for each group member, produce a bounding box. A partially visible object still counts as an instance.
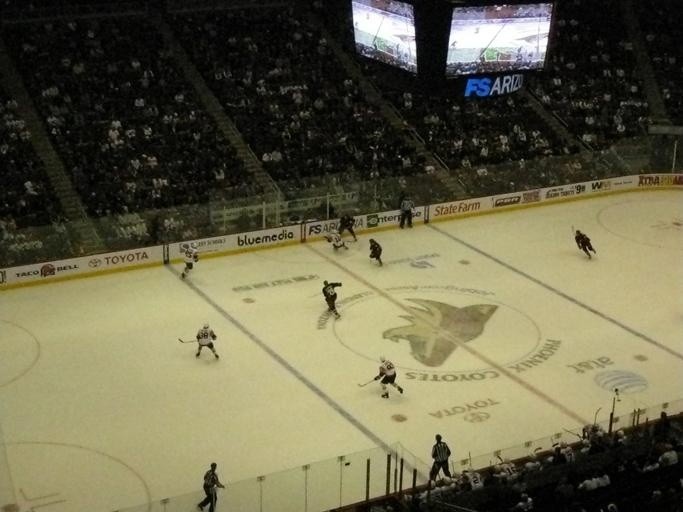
[358,380,375,387]
[179,339,198,343]
[571,225,576,237]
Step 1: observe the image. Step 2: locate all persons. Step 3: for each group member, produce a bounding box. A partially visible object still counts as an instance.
[321,280,341,319]
[195,462,224,512]
[193,323,219,360]
[374,356,402,397]
